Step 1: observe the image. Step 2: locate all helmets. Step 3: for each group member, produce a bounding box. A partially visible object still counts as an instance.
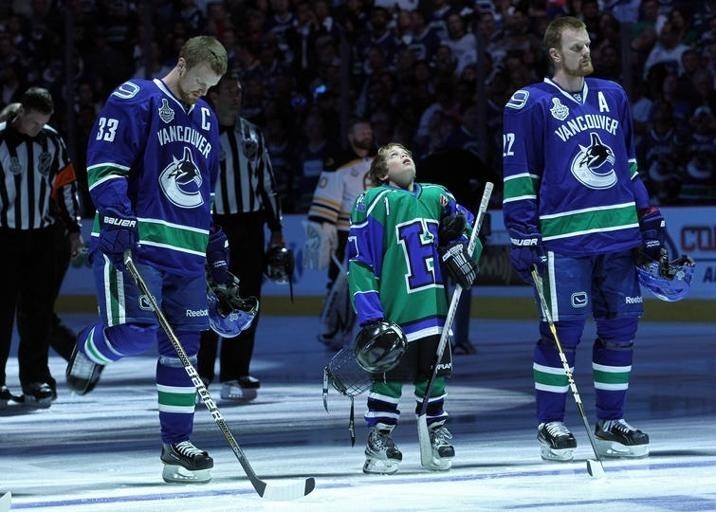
[262,246,294,286]
[635,254,695,303]
[328,319,408,397]
[206,272,259,339]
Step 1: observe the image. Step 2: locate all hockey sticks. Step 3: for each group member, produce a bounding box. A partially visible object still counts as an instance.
[417,182,494,466]
[532,264,606,477]
[125,251,315,499]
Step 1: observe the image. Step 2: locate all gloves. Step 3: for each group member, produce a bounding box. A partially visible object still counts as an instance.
[99,208,137,271]
[639,209,667,260]
[508,227,547,286]
[207,225,230,285]
[437,241,480,290]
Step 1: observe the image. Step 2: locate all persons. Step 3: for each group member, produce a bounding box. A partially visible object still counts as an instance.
[64,32,229,473]
[0,1,715,218]
[195,73,284,388]
[345,140,483,463]
[410,128,492,354]
[305,119,378,352]
[500,14,665,450]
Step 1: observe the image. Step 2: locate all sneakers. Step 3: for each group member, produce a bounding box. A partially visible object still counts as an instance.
[66,327,105,395]
[160,440,213,470]
[452,340,477,356]
[0,382,53,400]
[223,375,261,388]
[365,422,402,460]
[427,419,455,457]
[594,418,649,445]
[536,421,577,448]
